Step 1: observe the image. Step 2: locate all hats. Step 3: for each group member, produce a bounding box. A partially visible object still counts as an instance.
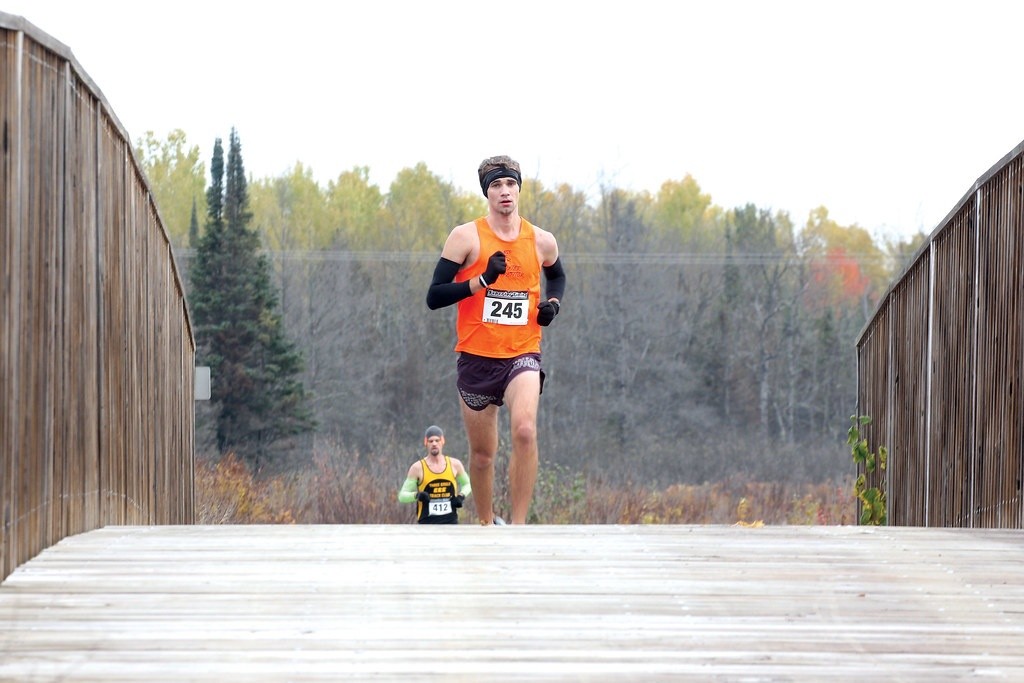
[424,425,442,438]
[479,156,521,197]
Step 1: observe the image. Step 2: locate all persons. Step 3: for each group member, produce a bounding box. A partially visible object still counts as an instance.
[425,156,566,525]
[398,426,472,524]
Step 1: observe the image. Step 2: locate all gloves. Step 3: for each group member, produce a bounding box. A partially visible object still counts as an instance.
[417,491,430,503]
[451,496,464,507]
[479,251,506,287]
[537,301,559,326]
